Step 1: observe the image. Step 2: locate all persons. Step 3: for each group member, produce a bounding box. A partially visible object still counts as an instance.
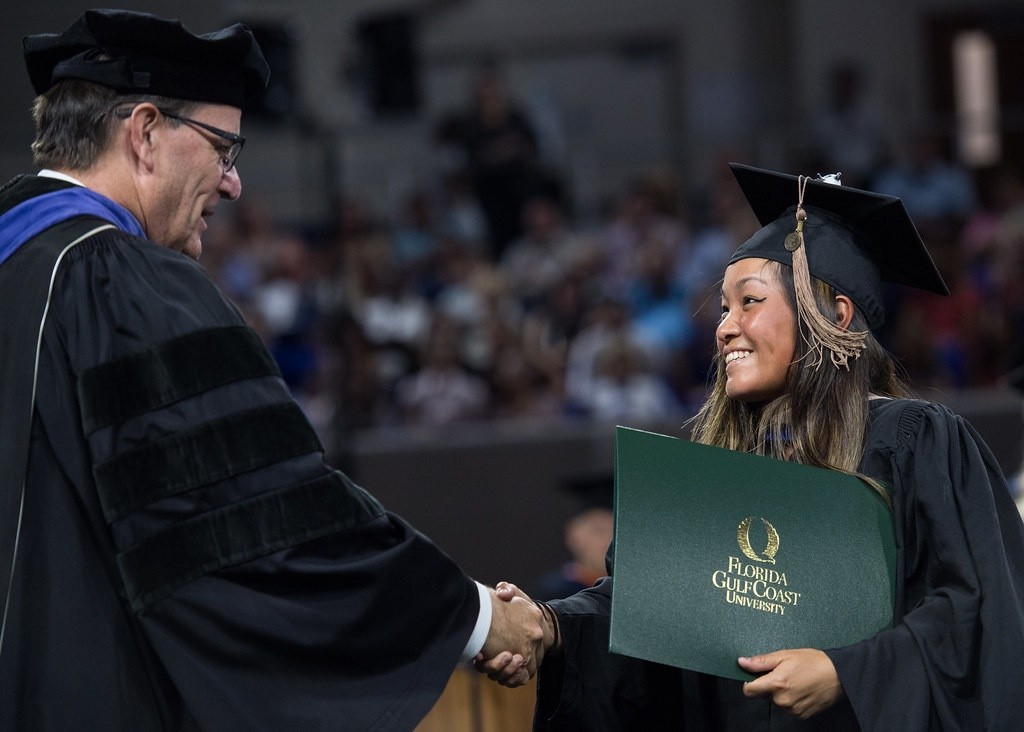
[475,163,1024,732]
[199,60,1024,598]
[0,0,543,732]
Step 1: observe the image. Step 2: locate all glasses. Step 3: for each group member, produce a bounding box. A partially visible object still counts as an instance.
[114,106,248,173]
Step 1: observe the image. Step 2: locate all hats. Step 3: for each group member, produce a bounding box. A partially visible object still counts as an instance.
[726,160,952,373]
[21,6,272,110]
[548,469,613,522]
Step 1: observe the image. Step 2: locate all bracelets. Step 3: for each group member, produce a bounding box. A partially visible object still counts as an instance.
[533,599,558,657]
